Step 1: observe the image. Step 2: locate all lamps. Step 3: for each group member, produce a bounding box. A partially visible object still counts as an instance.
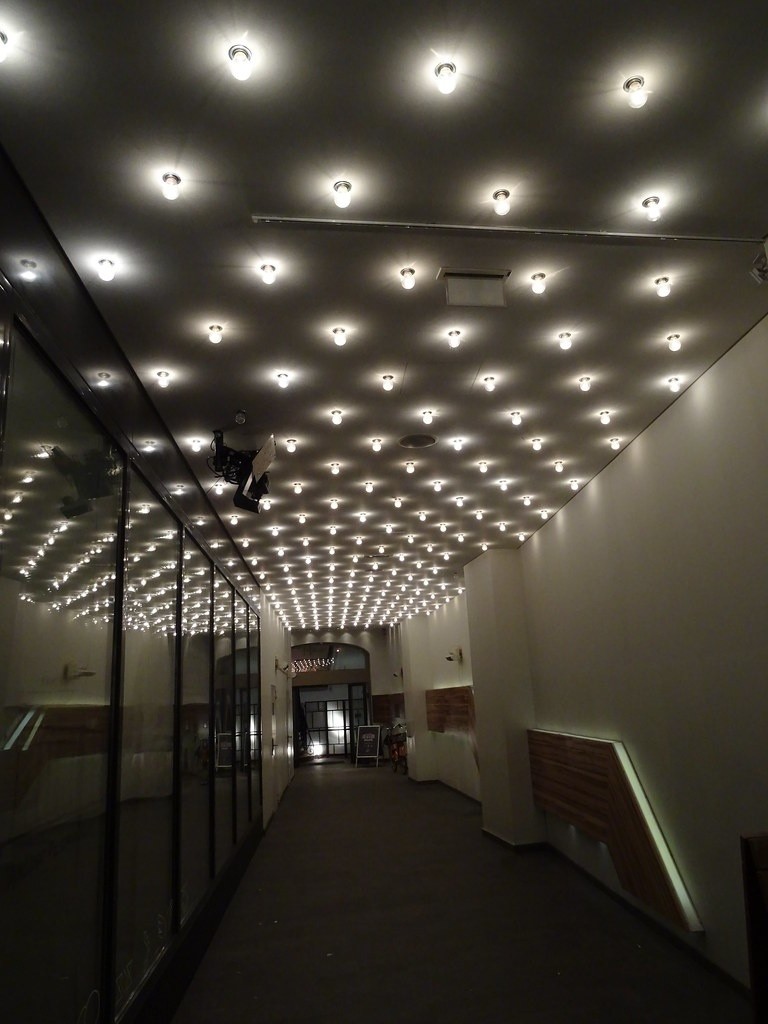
[444,647,460,664]
[393,669,402,679]
[274,658,297,679]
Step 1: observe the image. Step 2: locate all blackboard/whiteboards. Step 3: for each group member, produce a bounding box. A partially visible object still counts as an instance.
[219,734,233,765]
[356,725,380,758]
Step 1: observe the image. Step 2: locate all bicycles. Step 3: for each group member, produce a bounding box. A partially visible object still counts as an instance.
[384,723,408,777]
